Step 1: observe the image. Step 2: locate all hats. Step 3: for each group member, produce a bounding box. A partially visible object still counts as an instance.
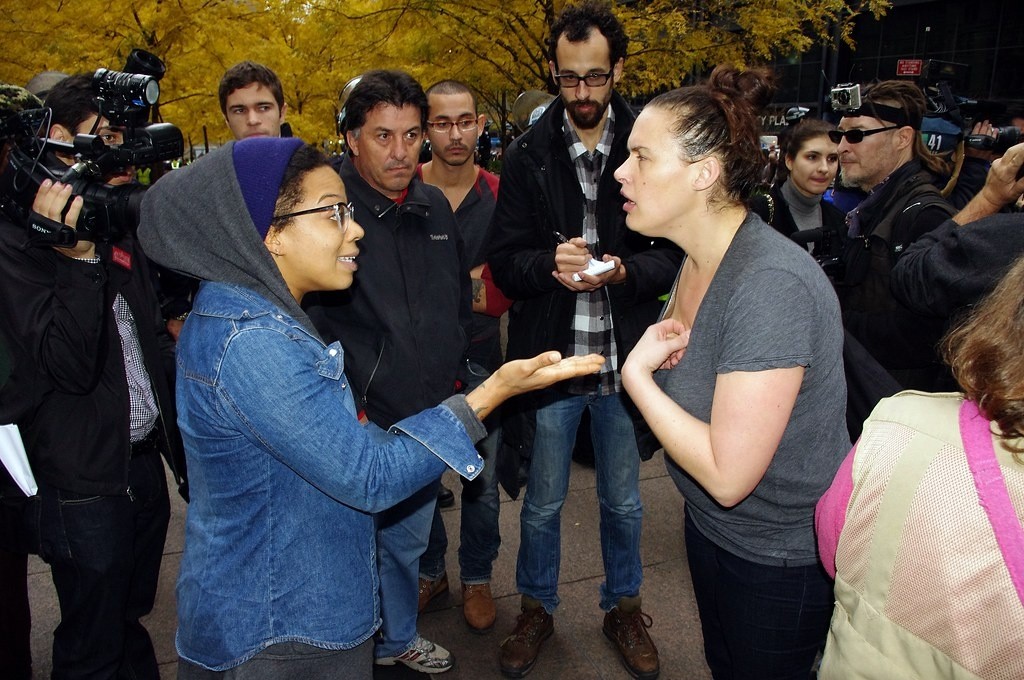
[234,135,303,243]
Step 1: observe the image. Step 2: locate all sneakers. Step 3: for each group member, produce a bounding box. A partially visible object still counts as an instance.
[417,571,448,614]
[375,636,455,673]
[499,596,554,678]
[602,595,660,680]
[460,581,496,634]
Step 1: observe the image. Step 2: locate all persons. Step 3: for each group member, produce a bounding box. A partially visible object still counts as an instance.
[413,81,510,630]
[219,61,283,142]
[752,77,1024,680]
[613,67,854,680]
[489,6,681,680]
[299,70,470,674]
[0,69,200,680]
[136,137,606,680]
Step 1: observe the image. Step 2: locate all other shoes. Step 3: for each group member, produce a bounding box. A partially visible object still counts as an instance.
[438,484,454,506]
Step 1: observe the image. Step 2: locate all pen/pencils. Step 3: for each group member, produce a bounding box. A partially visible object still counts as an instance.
[553,231,569,243]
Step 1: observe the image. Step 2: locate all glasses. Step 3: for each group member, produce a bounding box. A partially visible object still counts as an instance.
[553,65,615,88]
[827,126,900,144]
[272,202,355,234]
[426,118,478,134]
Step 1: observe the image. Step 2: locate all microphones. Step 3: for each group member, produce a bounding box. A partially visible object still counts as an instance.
[789,227,837,243]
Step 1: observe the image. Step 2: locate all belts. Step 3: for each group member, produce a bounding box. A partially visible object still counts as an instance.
[129,426,160,455]
